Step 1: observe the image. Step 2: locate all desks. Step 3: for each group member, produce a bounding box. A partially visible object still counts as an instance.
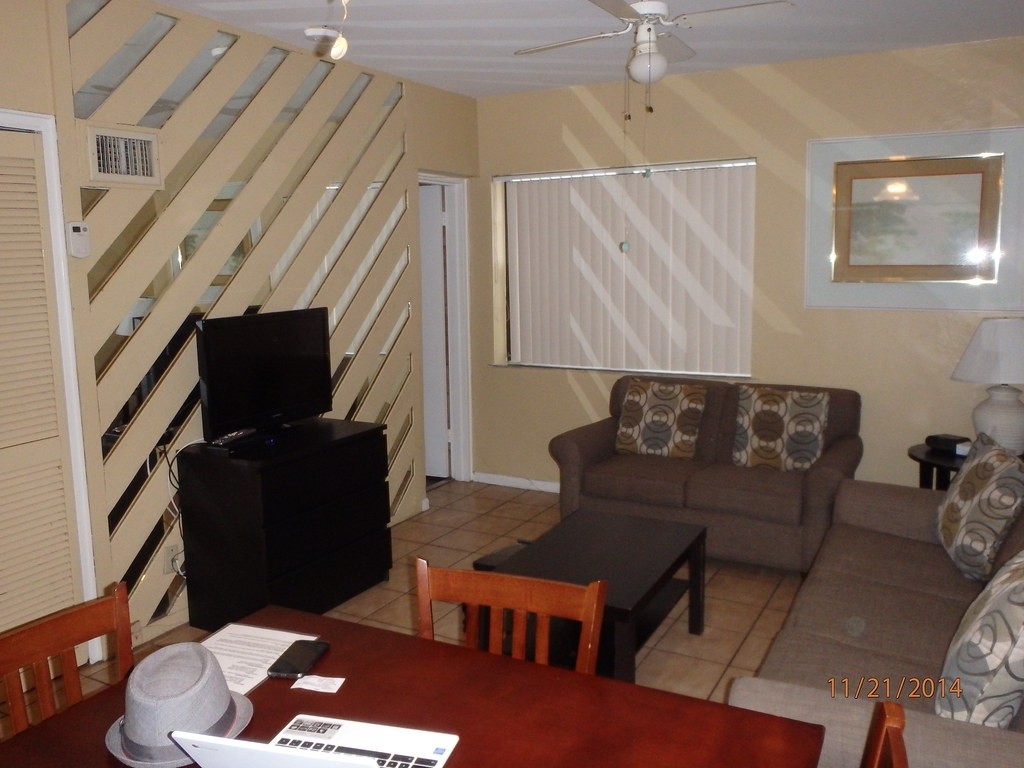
[1,606,828,768]
[909,443,967,492]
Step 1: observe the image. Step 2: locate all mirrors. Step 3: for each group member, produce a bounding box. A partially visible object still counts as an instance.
[830,156,1001,282]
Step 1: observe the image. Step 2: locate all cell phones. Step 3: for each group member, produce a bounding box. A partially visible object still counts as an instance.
[267,640,329,679]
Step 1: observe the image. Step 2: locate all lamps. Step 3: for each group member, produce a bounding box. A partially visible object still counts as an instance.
[624,23,667,121]
[331,0,349,61]
[873,178,920,202]
[948,317,1024,454]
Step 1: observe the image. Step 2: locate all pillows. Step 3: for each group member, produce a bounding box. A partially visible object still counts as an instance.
[611,378,709,459]
[729,385,831,470]
[932,552,1024,729]
[935,434,1024,579]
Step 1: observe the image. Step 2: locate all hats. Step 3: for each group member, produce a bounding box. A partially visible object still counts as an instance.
[105,642,254,768]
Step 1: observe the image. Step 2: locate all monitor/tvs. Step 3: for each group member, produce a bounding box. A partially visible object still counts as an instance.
[196,307,334,444]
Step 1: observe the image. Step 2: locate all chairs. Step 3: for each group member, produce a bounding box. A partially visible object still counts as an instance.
[413,559,609,679]
[857,701,909,767]
[1,579,133,744]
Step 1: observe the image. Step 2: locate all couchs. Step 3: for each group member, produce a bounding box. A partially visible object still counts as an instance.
[724,478,1024,768]
[548,375,865,574]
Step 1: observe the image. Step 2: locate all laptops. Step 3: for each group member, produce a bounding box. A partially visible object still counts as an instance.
[172,713,460,768]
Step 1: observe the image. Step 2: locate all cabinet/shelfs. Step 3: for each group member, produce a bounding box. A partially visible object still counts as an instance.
[176,418,391,635]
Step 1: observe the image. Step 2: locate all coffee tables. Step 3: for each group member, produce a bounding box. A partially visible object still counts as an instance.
[463,508,705,686]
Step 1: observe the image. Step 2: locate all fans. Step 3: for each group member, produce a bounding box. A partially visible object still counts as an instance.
[515,0,795,63]
